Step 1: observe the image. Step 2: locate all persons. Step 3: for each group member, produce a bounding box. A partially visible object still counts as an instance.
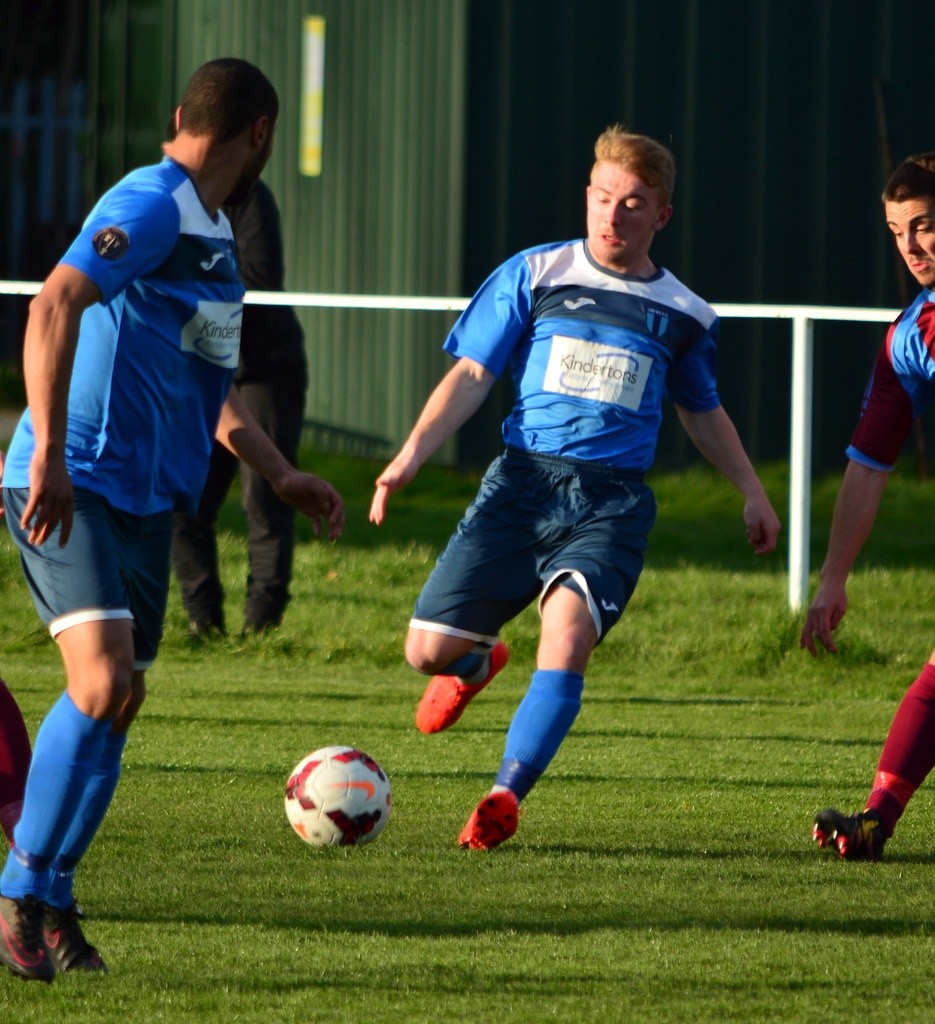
[0,57,343,986]
[369,134,782,851]
[0,680,33,850]
[797,153,935,854]
[167,176,310,645]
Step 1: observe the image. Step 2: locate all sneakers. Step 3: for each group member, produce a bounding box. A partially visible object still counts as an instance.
[189,606,226,653]
[0,892,55,985]
[810,807,888,862]
[0,800,21,846]
[41,899,108,976]
[415,640,509,734]
[454,793,518,852]
[241,618,258,639]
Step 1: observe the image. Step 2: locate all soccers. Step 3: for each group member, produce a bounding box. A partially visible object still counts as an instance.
[284,745,392,849]
